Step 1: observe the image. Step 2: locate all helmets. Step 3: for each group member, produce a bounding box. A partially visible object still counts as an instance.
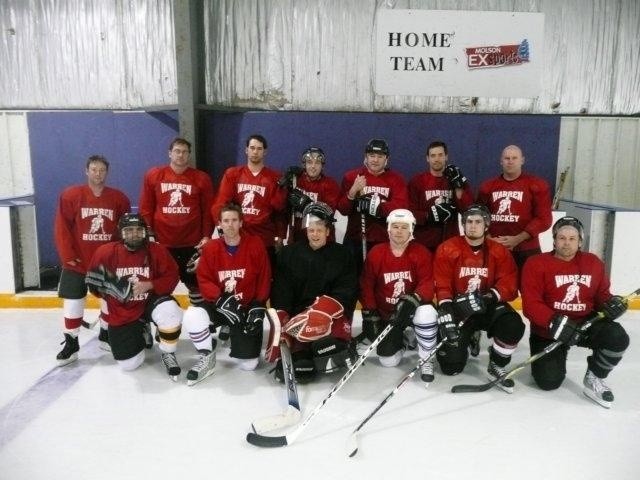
[552,213,584,242]
[461,204,492,228]
[116,213,149,249]
[300,200,337,230]
[364,138,392,158]
[302,147,326,165]
[385,209,417,234]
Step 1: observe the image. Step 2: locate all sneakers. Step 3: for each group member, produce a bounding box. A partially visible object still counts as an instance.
[421,362,435,382]
[142,320,154,350]
[55,332,80,362]
[160,353,181,376]
[468,331,482,357]
[97,326,111,346]
[217,324,231,342]
[583,369,615,402]
[487,358,516,387]
[404,327,419,351]
[186,349,219,381]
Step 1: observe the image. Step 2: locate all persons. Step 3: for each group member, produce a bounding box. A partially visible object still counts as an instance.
[520,217,629,409]
[337,139,410,262]
[361,209,438,388]
[181,203,271,387]
[272,201,365,385]
[86,213,182,383]
[285,144,342,241]
[432,202,525,395]
[138,137,219,342]
[54,155,132,368]
[476,145,553,267]
[211,132,289,259]
[410,140,476,252]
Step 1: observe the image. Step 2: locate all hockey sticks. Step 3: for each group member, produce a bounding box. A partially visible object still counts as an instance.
[247,323,395,448]
[355,171,377,342]
[346,316,468,457]
[453,288,640,393]
[251,344,301,434]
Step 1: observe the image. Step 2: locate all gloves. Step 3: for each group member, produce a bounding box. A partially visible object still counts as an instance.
[426,200,458,226]
[356,194,383,220]
[545,311,586,349]
[216,292,246,328]
[455,287,498,331]
[289,191,311,214]
[242,298,266,338]
[84,264,133,303]
[436,299,464,349]
[443,164,469,188]
[360,308,387,346]
[264,309,292,365]
[600,296,629,321]
[389,292,421,335]
[284,294,347,346]
